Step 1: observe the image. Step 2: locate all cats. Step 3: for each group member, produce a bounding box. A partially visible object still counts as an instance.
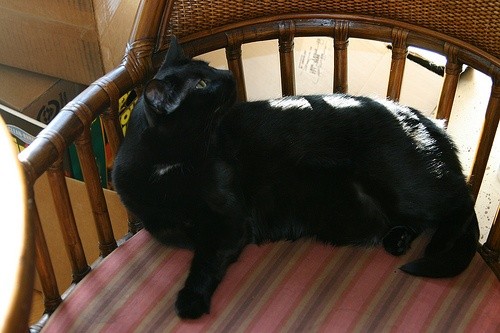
[111,36,480,320]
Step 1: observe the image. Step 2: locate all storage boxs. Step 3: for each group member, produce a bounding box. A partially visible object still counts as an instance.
[0,0,140,84]
[0,65,81,128]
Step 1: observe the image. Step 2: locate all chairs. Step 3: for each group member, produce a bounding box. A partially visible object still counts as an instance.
[3,0,500,333]
